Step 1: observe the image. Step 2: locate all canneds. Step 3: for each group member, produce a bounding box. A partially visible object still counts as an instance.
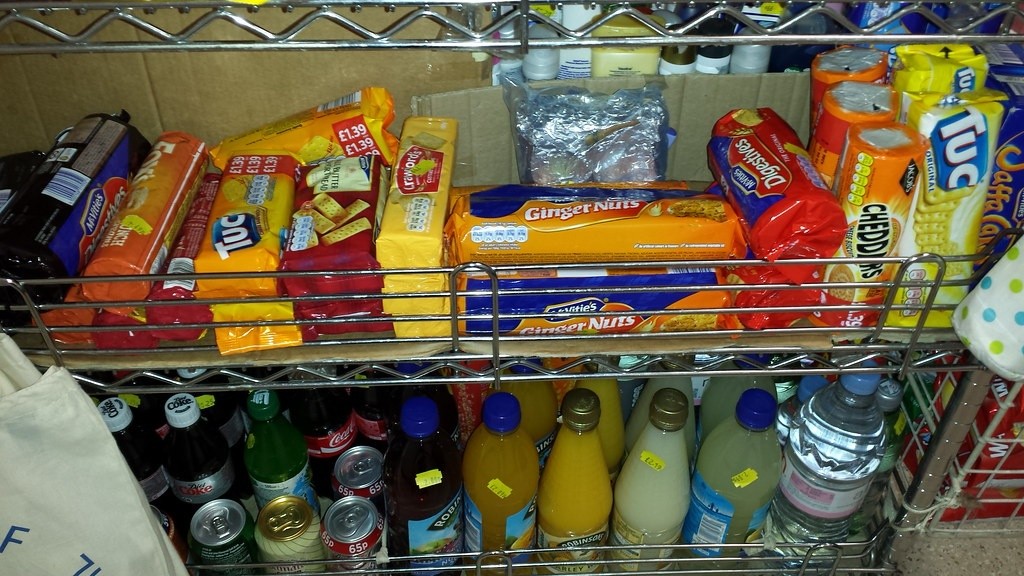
[150,446,390,576]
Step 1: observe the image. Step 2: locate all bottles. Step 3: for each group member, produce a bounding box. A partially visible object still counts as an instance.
[847,379,907,539]
[494,356,557,480]
[605,388,690,576]
[464,392,539,575]
[291,371,360,502]
[381,396,464,576]
[178,369,246,475]
[349,365,394,456]
[758,359,887,576]
[575,354,624,492]
[776,374,830,450]
[246,390,321,519]
[161,393,239,520]
[537,388,612,576]
[98,397,193,536]
[676,388,782,576]
[689,353,778,484]
[113,370,172,440]
[621,355,695,476]
[396,363,461,449]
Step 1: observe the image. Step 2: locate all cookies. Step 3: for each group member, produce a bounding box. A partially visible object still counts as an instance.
[291,192,373,250]
[914,147,976,277]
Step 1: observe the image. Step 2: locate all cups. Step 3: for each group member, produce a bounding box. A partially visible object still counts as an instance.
[237,366,291,436]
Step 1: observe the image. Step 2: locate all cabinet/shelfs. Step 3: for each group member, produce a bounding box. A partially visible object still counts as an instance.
[1,0,1024,576]
